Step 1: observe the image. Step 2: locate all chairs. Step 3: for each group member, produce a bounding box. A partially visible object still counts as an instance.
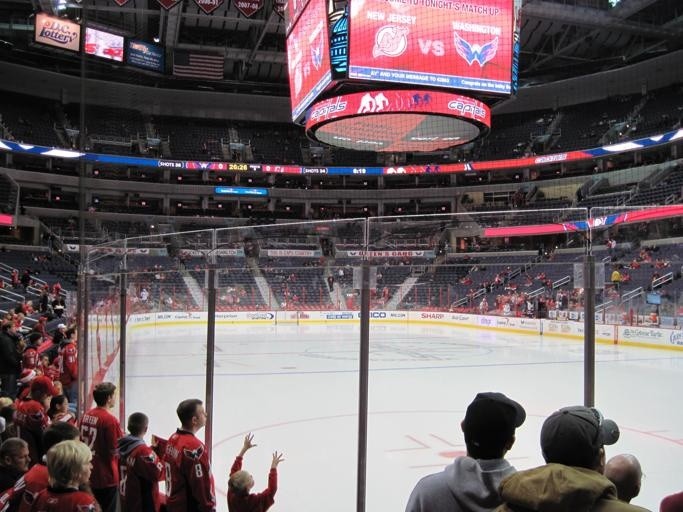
[208,242,362,311]
[161,115,386,166]
[85,241,208,314]
[610,86,682,144]
[0,89,78,152]
[0,249,79,340]
[451,241,586,322]
[593,241,683,328]
[458,96,610,163]
[78,103,161,159]
[461,162,683,236]
[386,146,458,168]
[369,241,451,312]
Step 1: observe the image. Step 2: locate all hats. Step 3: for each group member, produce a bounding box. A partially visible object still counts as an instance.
[39,317,47,321]
[30,375,60,400]
[539,405,620,466]
[18,368,37,383]
[57,323,66,329]
[463,391,527,448]
